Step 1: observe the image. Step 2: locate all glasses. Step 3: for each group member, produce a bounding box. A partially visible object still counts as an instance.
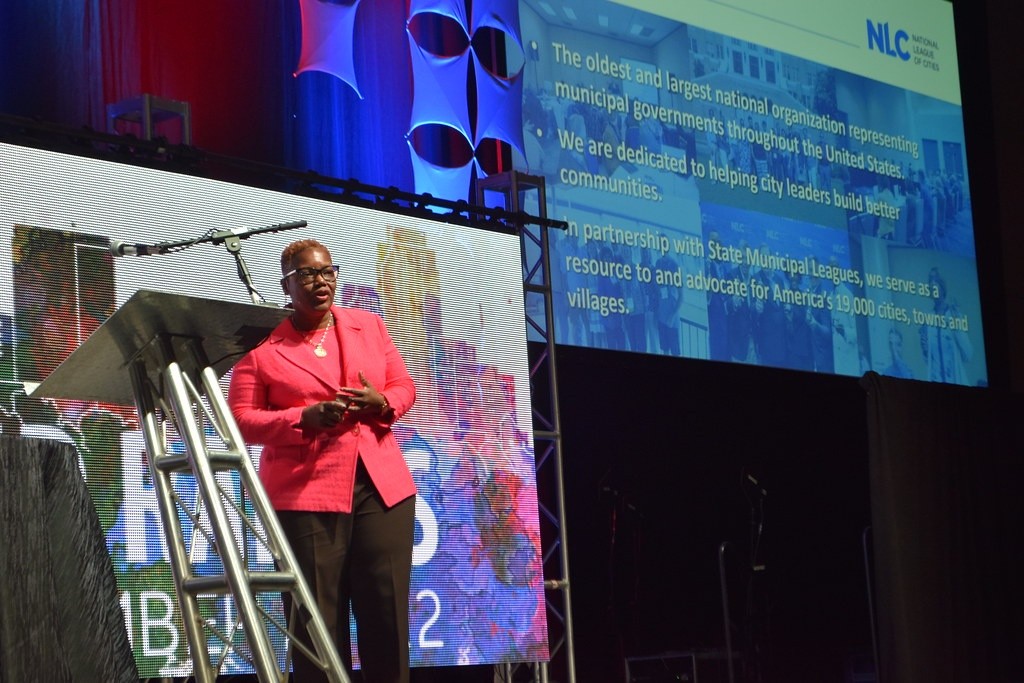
[281,265,340,285]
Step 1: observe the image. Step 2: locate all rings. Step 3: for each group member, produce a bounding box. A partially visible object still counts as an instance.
[327,419,329,425]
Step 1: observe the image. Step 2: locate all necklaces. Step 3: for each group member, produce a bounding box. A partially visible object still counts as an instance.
[292,312,333,357]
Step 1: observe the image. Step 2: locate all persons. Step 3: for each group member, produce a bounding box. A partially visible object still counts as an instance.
[521,68,972,387]
[229,241,417,683]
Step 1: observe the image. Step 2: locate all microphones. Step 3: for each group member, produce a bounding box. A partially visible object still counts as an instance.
[603,485,637,512]
[111,239,166,257]
[748,474,768,495]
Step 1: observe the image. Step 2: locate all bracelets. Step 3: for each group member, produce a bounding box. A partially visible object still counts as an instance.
[381,401,387,409]
